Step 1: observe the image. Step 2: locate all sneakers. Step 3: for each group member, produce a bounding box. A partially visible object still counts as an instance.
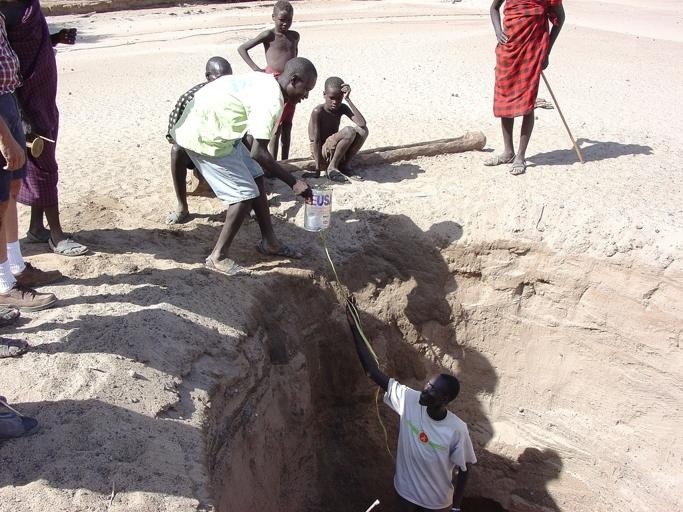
[0,415,40,441]
[0,262,62,358]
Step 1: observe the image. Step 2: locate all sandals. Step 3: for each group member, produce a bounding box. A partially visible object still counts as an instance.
[256,239,303,259]
[205,254,253,277]
[24,230,89,256]
[483,153,527,175]
[165,211,190,225]
[324,167,365,184]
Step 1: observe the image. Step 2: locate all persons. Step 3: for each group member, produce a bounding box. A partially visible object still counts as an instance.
[236,0,300,161]
[0,307,29,358]
[1,1,89,255]
[171,57,318,277]
[307,78,368,184]
[345,294,477,511]
[0,394,40,438]
[166,56,233,222]
[485,1,565,175]
[1,13,64,313]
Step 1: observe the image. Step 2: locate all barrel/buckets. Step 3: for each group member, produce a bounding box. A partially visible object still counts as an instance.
[303,185,333,233]
